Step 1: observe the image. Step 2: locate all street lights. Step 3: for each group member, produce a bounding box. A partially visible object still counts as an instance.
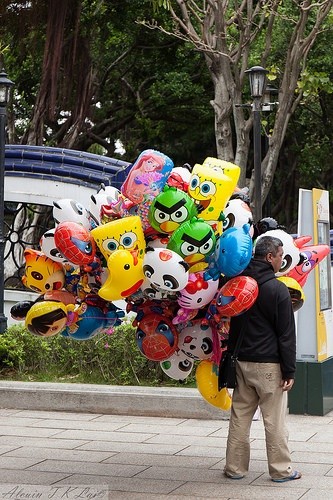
[0,65,16,334]
[236,66,279,222]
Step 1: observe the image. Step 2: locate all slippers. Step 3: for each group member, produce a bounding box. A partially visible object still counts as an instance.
[273,471,302,482]
[227,472,243,478]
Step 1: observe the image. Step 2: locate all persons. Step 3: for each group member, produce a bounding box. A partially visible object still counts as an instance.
[222,235,303,483]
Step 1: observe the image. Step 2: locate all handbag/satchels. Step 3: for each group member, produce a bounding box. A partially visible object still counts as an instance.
[218,350,237,391]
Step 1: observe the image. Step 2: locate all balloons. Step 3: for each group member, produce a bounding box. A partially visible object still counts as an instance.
[10,147,330,413]
